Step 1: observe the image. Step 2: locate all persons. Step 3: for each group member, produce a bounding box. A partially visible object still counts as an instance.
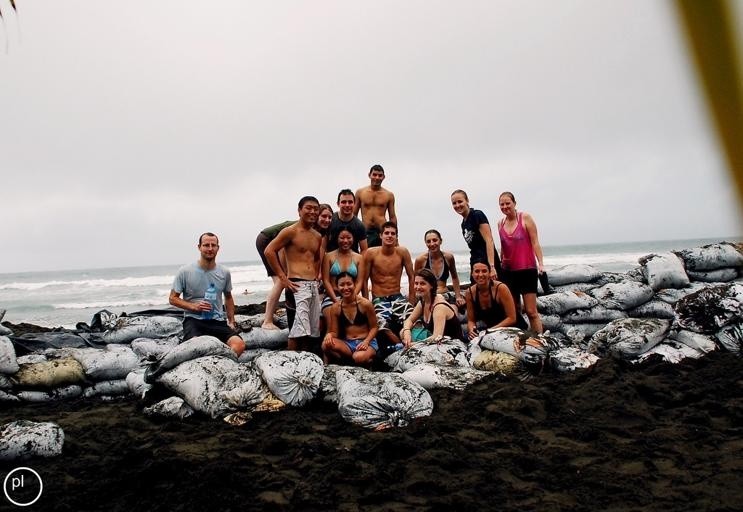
[414,230,467,316]
[451,189,503,287]
[466,258,528,341]
[256,164,415,368]
[169,233,246,359]
[498,192,543,337]
[399,268,465,350]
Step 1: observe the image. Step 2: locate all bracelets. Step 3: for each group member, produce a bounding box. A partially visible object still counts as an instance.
[489,265,494,267]
[228,321,238,326]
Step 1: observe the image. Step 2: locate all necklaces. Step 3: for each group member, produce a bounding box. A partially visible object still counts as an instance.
[477,287,490,297]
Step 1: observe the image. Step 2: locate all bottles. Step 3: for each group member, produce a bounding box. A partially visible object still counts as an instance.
[202,283,217,321]
[385,342,403,352]
